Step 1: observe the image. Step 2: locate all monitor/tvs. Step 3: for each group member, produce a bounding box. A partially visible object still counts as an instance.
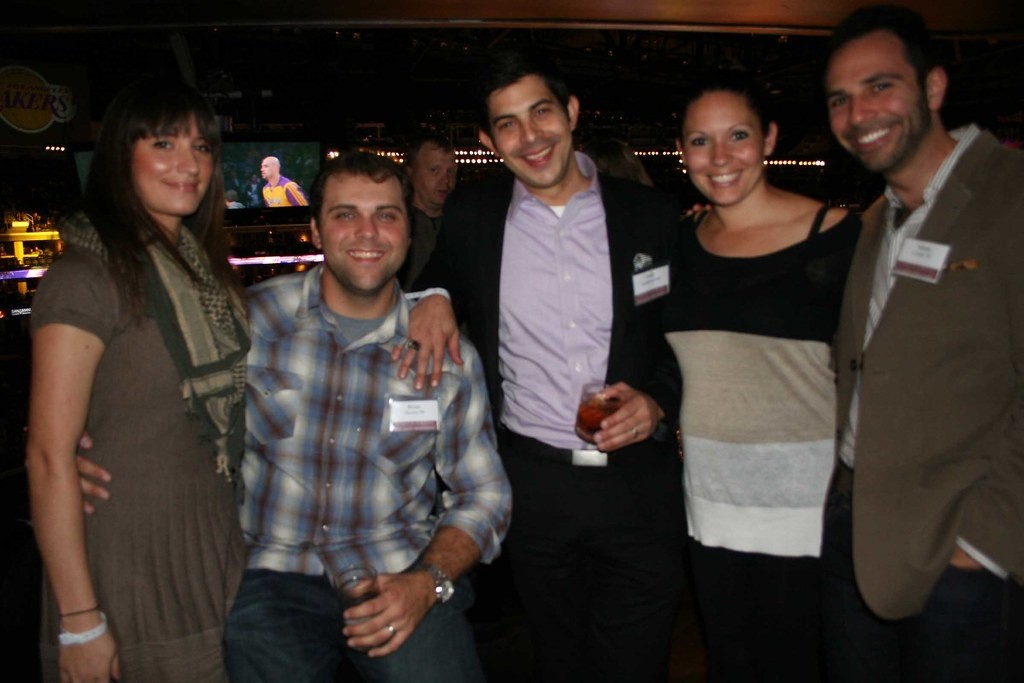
[211,128,330,224]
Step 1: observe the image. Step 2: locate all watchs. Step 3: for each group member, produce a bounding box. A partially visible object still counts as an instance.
[413,563,454,603]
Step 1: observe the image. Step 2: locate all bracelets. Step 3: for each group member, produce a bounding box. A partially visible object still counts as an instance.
[60,605,99,617]
[58,612,107,645]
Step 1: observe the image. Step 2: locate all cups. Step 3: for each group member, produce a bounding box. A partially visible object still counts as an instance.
[334,562,382,652]
[575,382,627,444]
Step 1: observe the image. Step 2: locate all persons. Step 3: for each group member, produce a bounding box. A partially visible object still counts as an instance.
[25,52,688,683]
[246,174,261,197]
[261,155,308,206]
[686,1,1024,683]
[662,77,860,683]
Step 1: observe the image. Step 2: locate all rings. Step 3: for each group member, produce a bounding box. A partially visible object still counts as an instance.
[408,341,419,350]
[386,624,396,634]
[631,429,638,438]
